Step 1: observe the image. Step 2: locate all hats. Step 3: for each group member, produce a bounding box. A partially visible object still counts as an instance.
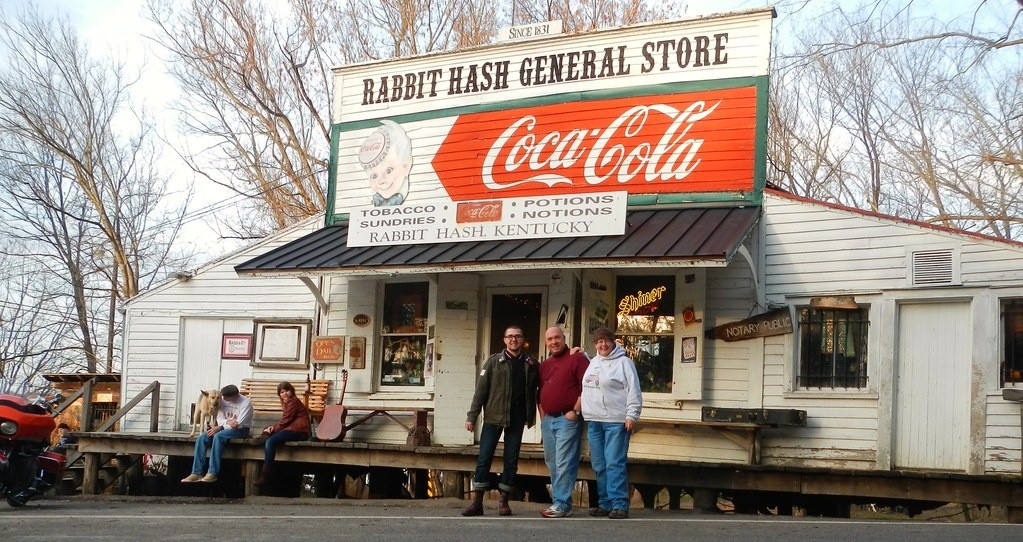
[58,423,70,429]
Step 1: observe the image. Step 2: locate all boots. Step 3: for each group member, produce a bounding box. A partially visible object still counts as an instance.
[461,490,484,516]
[253,462,279,487]
[500,491,512,515]
[250,432,270,445]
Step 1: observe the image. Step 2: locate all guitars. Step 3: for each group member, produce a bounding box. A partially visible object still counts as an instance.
[316,368,349,441]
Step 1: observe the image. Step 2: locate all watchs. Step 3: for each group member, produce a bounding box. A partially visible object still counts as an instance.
[572,408,580,417]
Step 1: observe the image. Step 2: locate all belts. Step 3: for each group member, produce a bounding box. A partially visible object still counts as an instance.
[550,410,569,418]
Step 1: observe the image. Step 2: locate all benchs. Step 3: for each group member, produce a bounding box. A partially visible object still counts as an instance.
[635,418,779,465]
[343,405,434,447]
[239,377,332,424]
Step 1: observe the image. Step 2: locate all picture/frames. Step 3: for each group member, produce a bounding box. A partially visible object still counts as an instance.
[221,332,254,360]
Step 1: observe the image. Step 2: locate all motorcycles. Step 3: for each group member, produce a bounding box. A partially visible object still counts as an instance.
[0,376,67,507]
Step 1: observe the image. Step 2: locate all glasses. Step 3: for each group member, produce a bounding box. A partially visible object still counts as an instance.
[504,334,523,341]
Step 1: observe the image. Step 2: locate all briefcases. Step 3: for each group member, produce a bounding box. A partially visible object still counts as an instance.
[38,451,67,476]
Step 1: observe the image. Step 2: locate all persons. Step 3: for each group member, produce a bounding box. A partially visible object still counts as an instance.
[181,385,252,482]
[460,325,540,515]
[580,326,643,519]
[252,381,312,486]
[536,325,590,517]
[29,422,78,498]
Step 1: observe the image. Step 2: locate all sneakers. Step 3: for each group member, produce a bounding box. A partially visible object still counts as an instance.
[181,473,204,482]
[540,504,573,518]
[201,474,217,483]
[608,510,628,519]
[590,508,610,516]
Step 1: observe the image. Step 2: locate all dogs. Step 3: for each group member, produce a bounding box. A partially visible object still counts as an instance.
[190,389,223,437]
